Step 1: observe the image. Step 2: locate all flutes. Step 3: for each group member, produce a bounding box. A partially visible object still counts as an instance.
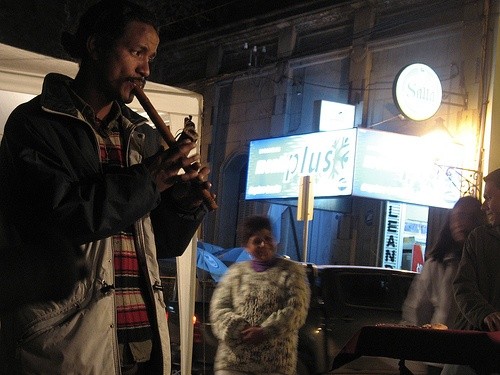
[131,82,219,213]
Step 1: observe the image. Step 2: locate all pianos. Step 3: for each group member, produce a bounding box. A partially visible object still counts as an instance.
[354,324,500,368]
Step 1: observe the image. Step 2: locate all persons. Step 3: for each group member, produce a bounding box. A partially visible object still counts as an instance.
[399,169,500,375]
[0,0,216,375]
[210,214,311,375]
